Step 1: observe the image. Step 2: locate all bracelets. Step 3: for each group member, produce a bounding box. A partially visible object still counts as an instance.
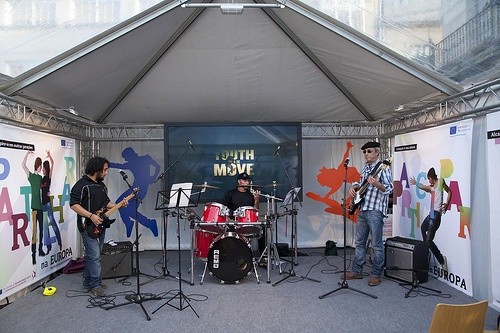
[90,214,93,218]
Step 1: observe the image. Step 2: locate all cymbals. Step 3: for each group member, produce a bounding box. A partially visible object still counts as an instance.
[237,184,262,187]
[265,183,284,187]
[192,184,220,189]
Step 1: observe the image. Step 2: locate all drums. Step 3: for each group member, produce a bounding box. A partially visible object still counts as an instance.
[234,205,261,237]
[194,229,218,262]
[198,201,230,234]
[206,232,255,284]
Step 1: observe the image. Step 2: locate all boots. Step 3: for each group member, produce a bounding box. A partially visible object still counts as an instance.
[39,243,46,257]
[32,244,36,264]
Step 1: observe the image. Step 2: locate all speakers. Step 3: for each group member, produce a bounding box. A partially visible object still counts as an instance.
[100,241,133,279]
[383,236,429,284]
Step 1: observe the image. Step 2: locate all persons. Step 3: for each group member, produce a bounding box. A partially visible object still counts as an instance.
[69,157,128,297]
[340,141,395,285]
[222,173,261,232]
[22,149,62,265]
[410,168,452,271]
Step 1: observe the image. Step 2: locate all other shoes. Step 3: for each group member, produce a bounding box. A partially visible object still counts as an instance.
[441,255,448,271]
[47,243,52,255]
[340,271,362,279]
[59,240,62,250]
[83,287,90,293]
[92,286,104,296]
[368,277,380,286]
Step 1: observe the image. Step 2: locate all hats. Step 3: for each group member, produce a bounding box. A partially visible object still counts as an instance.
[361,141,380,150]
[238,172,253,179]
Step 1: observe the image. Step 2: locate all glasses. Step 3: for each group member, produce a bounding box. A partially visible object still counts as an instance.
[362,150,371,153]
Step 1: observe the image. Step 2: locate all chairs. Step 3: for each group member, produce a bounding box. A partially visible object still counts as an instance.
[428,300,488,333]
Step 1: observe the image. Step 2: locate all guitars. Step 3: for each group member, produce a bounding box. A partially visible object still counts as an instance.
[348,158,392,215]
[82,187,141,239]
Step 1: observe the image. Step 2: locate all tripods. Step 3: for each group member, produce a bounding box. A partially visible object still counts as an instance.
[318,167,377,299]
[105,147,202,321]
[390,267,441,298]
[271,152,321,287]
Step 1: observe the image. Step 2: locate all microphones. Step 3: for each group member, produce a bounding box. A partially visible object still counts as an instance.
[344,158,350,167]
[273,146,280,156]
[382,266,398,270]
[119,169,128,179]
[189,140,195,151]
[112,267,116,271]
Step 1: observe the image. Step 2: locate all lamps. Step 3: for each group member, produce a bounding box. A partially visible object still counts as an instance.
[221,0,243,13]
